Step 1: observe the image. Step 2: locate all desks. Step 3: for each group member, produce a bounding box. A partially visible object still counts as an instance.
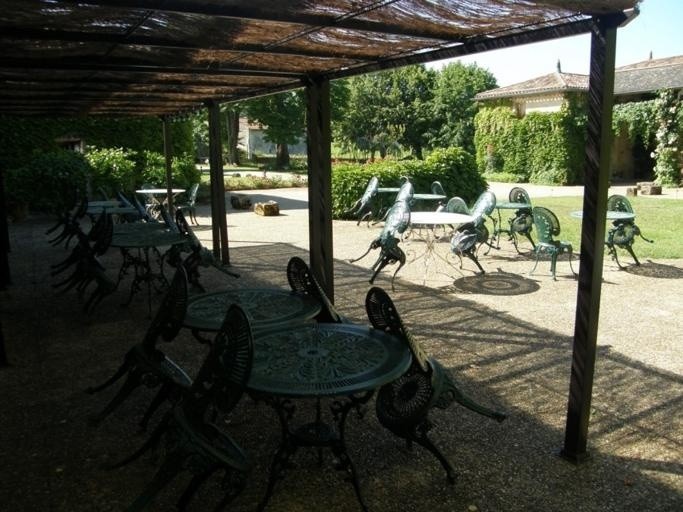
[173,287,323,425]
[228,325,414,512]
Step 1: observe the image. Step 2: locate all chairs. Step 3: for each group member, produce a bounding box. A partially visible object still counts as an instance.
[365,286,509,486]
[102,302,253,511]
[286,256,349,324]
[43,177,241,320]
[90,265,192,420]
